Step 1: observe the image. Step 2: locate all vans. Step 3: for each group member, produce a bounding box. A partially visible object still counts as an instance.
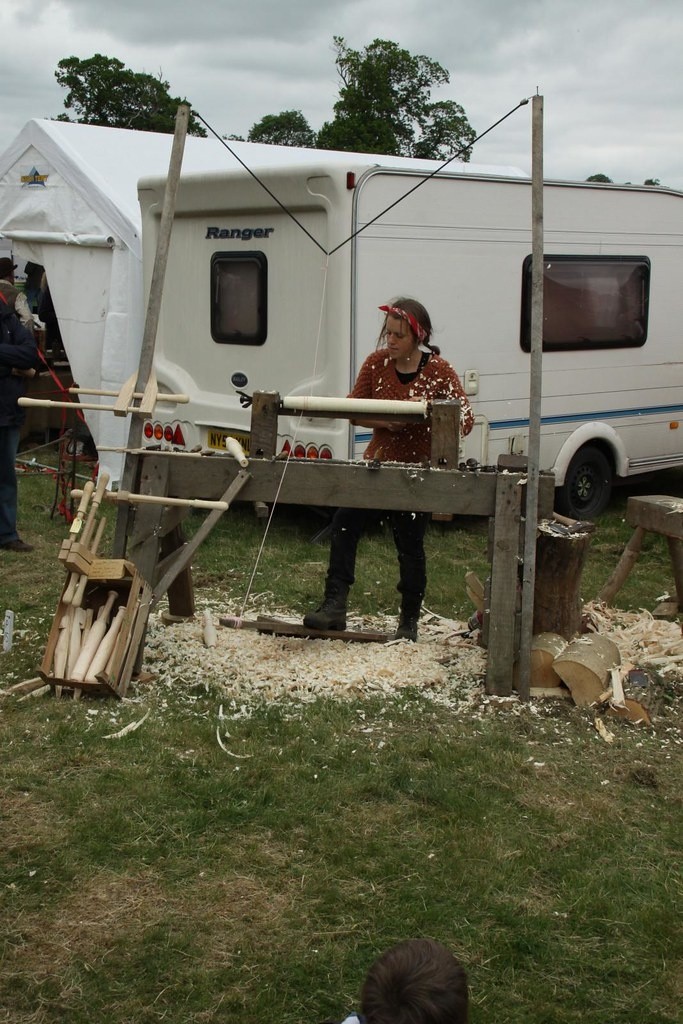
[133,163,683,521]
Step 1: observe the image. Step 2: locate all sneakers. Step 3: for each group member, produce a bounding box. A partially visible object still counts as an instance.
[0,540,33,552]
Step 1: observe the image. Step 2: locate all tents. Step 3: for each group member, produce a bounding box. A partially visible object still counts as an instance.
[0,117,531,488]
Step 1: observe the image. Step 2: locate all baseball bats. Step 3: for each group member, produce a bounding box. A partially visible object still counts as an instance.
[50,586,143,704]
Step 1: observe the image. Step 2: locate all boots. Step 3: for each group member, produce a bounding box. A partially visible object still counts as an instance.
[303,578,350,630]
[394,604,419,644]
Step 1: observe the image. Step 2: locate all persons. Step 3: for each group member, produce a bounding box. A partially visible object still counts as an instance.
[339,939,467,1024]
[302,296,474,642]
[0,258,66,549]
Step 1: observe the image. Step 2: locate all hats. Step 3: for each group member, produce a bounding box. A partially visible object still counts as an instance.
[0,257,18,278]
